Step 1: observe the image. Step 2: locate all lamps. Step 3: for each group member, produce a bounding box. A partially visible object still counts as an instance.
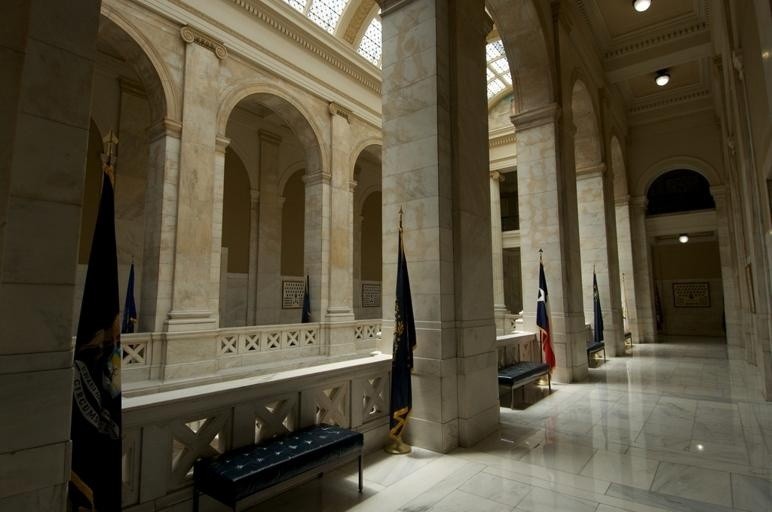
[679,234,689,243]
[655,70,671,86]
[632,0,652,13]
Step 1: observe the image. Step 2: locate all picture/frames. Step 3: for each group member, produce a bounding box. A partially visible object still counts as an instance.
[282,281,305,309]
[672,282,711,308]
[362,284,381,307]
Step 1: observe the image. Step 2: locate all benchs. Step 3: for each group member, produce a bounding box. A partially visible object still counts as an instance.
[624,332,632,349]
[586,341,606,367]
[194,423,363,512]
[499,361,551,410]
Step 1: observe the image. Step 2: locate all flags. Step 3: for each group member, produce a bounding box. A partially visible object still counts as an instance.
[535,261,556,384]
[300,273,312,323]
[58,152,123,511]
[591,270,605,343]
[387,222,419,445]
[654,278,664,331]
[620,278,631,334]
[120,261,138,364]
